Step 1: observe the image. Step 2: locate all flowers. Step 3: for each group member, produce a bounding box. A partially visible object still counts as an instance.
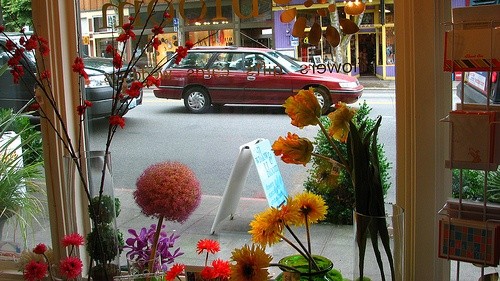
[0,0,395,281]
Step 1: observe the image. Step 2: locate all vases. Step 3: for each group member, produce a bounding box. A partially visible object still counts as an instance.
[274,255,335,281]
[130,272,164,281]
[63,151,120,281]
[354,203,403,281]
[127,249,161,275]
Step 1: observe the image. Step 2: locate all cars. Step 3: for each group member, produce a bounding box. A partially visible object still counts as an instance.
[0,31,143,132]
[154,46,364,116]
[456,69,500,105]
